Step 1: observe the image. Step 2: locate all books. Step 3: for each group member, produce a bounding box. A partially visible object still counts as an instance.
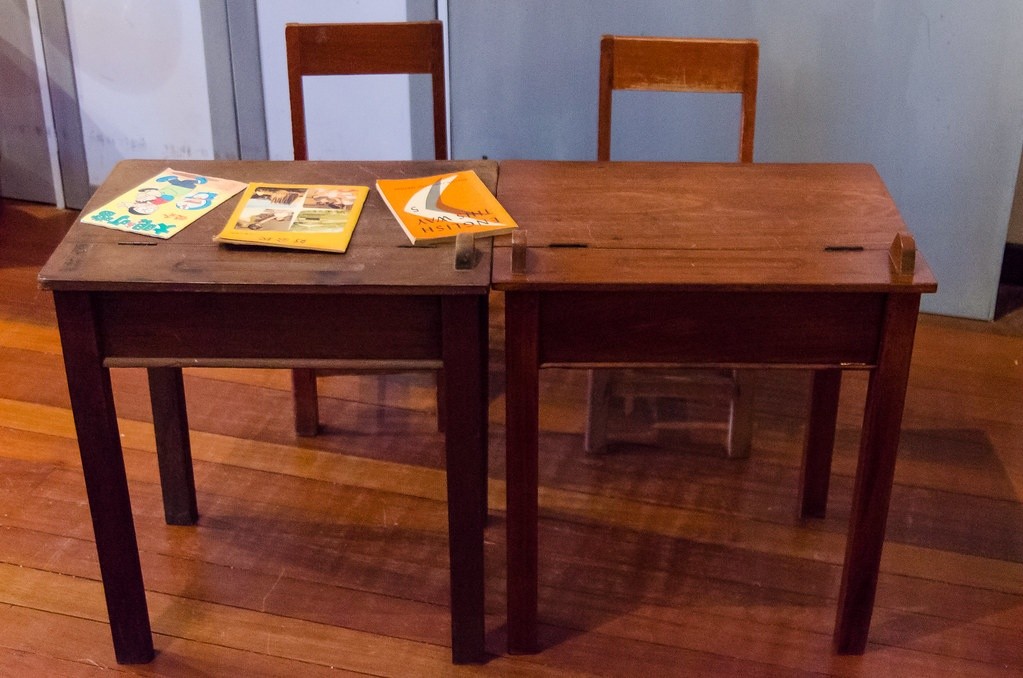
[79,166,248,240]
[213,179,371,255]
[376,169,519,245]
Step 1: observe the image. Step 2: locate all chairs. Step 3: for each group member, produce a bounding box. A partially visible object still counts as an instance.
[283,21,446,431]
[583,33,758,459]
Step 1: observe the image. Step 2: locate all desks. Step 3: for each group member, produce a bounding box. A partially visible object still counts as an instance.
[492,158,937,655]
[38,157,498,665]
